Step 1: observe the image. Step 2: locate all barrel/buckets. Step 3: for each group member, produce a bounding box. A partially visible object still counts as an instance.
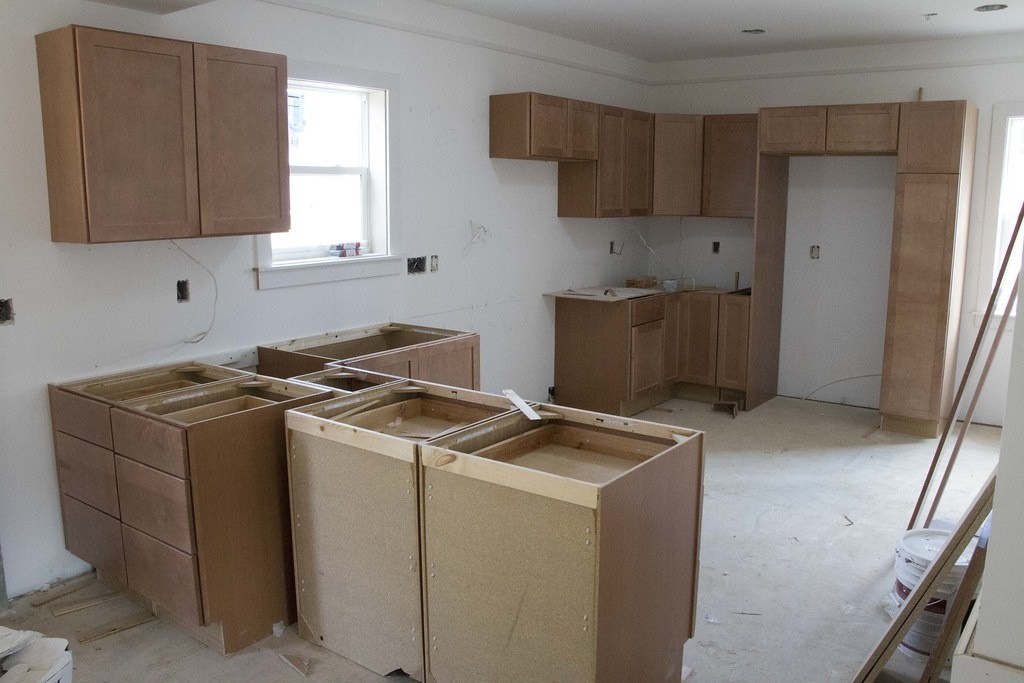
[892,528,982,661]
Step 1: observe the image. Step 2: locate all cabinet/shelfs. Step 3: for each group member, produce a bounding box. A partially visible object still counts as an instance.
[257,323,481,390]
[558,105,655,218]
[419,401,705,683]
[490,91,599,162]
[555,286,752,417]
[653,113,704,216]
[702,114,758,218]
[826,103,900,156]
[34,25,291,244]
[284,379,535,683]
[877,100,979,438]
[760,105,827,157]
[49,359,333,655]
